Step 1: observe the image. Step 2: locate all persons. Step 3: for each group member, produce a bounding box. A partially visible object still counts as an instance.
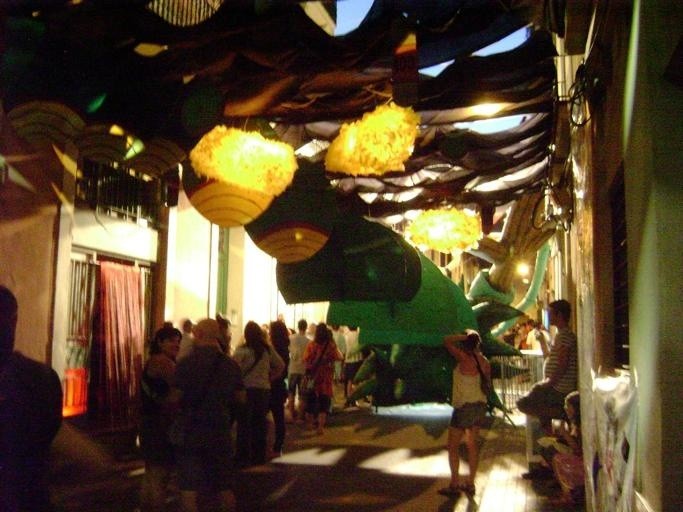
[514,298,578,480]
[0,283,66,511]
[137,315,367,512]
[548,391,586,505]
[437,328,492,499]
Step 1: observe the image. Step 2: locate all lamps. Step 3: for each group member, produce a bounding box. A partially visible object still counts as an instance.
[244,161,337,263]
[403,193,484,255]
[189,114,298,197]
[322,90,419,183]
[180,158,275,229]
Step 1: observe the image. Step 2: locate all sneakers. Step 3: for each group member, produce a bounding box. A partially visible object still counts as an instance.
[439,481,476,498]
